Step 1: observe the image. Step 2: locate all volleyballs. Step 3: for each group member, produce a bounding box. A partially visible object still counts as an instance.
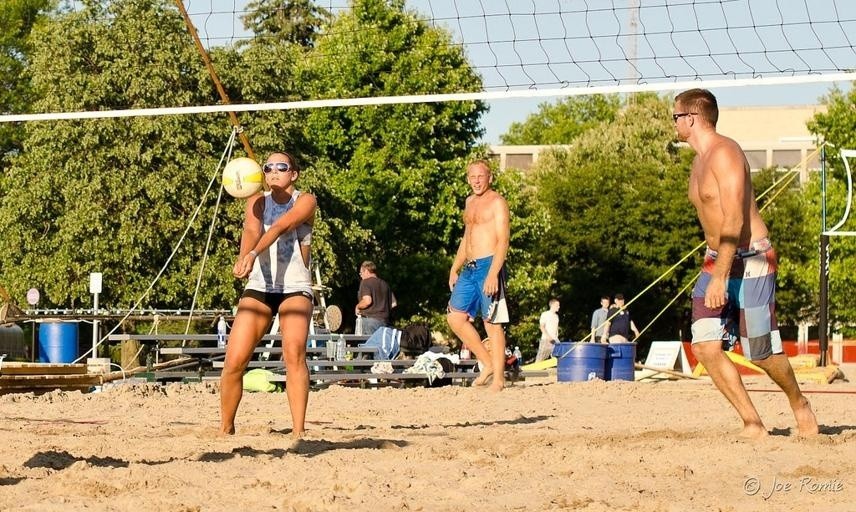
[223,157,263,198]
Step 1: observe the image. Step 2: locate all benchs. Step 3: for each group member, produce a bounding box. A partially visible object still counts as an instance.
[108,334,551,387]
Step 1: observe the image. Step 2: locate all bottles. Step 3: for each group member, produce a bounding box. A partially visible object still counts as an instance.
[513,346,523,365]
[217,316,227,349]
[336,333,353,373]
[354,313,363,336]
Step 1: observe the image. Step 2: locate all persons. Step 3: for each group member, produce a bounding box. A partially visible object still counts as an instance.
[601,294,641,342]
[198,151,317,442]
[673,88,820,441]
[535,298,561,363]
[590,296,620,343]
[354,260,399,388]
[447,159,511,403]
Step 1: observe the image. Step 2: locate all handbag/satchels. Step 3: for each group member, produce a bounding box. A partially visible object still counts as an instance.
[367,321,432,360]
[242,369,283,393]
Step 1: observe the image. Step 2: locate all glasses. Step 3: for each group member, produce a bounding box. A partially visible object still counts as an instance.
[262,162,290,173]
[674,113,696,122]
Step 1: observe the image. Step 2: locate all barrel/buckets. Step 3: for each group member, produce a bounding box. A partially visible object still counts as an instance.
[606,342,637,380]
[552,342,608,382]
[38,322,79,363]
[0,323,24,362]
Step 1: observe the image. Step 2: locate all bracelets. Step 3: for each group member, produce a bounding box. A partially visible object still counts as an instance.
[248,249,258,258]
